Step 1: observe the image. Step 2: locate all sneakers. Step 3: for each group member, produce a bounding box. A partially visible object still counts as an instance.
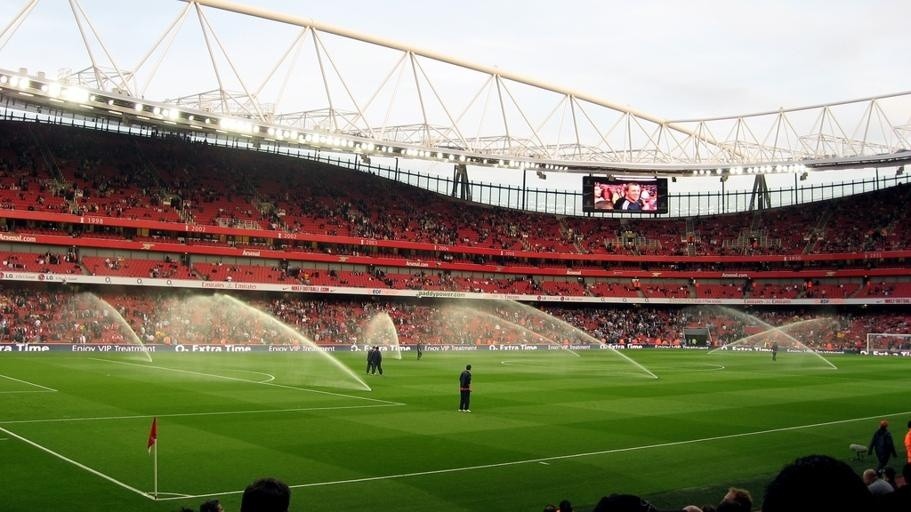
[458,409,472,412]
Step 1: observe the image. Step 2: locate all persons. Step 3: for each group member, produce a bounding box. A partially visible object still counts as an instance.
[1,119,911,354]
[181,478,292,512]
[366,346,383,374]
[762,456,874,512]
[545,488,754,512]
[864,419,911,512]
[458,361,474,410]
